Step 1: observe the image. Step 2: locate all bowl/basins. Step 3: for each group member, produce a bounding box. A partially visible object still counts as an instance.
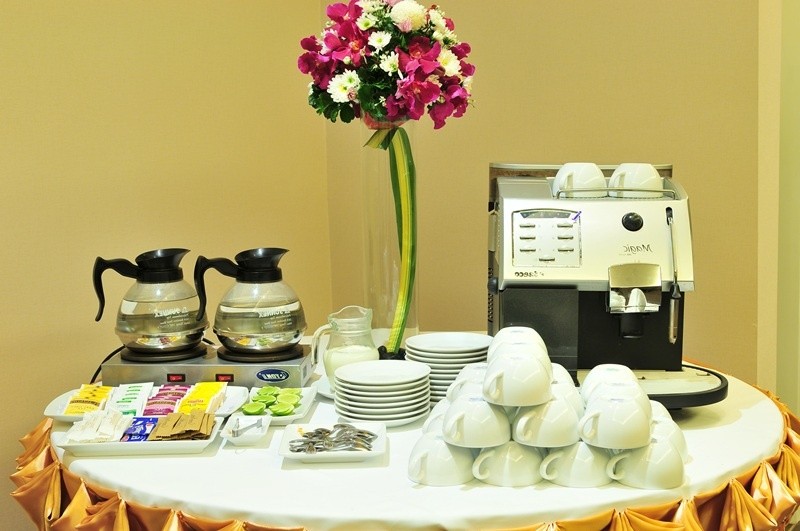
[220,415,272,446]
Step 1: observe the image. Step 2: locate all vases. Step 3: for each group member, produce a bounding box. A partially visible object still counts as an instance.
[361,100,419,361]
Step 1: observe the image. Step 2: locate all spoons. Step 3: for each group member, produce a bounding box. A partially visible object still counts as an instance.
[289,423,376,452]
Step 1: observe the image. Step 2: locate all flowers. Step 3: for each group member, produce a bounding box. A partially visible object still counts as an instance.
[299,0,477,129]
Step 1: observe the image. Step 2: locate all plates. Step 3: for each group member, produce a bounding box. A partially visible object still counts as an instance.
[42,387,317,457]
[312,376,334,400]
[283,424,386,463]
[334,332,494,428]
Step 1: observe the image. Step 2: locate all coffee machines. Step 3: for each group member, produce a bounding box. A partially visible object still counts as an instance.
[487,162,730,411]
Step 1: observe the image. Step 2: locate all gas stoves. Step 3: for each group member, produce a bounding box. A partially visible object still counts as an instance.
[100,343,318,388]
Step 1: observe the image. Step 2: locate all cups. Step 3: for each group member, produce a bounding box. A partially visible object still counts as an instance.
[409,325,686,490]
[553,162,607,198]
[609,163,664,198]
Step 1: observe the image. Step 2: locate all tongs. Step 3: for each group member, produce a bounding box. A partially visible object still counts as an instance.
[231,417,264,437]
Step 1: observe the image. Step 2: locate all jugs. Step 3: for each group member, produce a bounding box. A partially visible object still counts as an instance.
[310,306,380,393]
[194,247,308,354]
[93,248,211,353]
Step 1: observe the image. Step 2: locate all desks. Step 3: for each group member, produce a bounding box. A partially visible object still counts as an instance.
[10,328,800,531]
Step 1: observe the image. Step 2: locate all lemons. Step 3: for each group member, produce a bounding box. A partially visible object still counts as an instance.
[241,385,301,415]
